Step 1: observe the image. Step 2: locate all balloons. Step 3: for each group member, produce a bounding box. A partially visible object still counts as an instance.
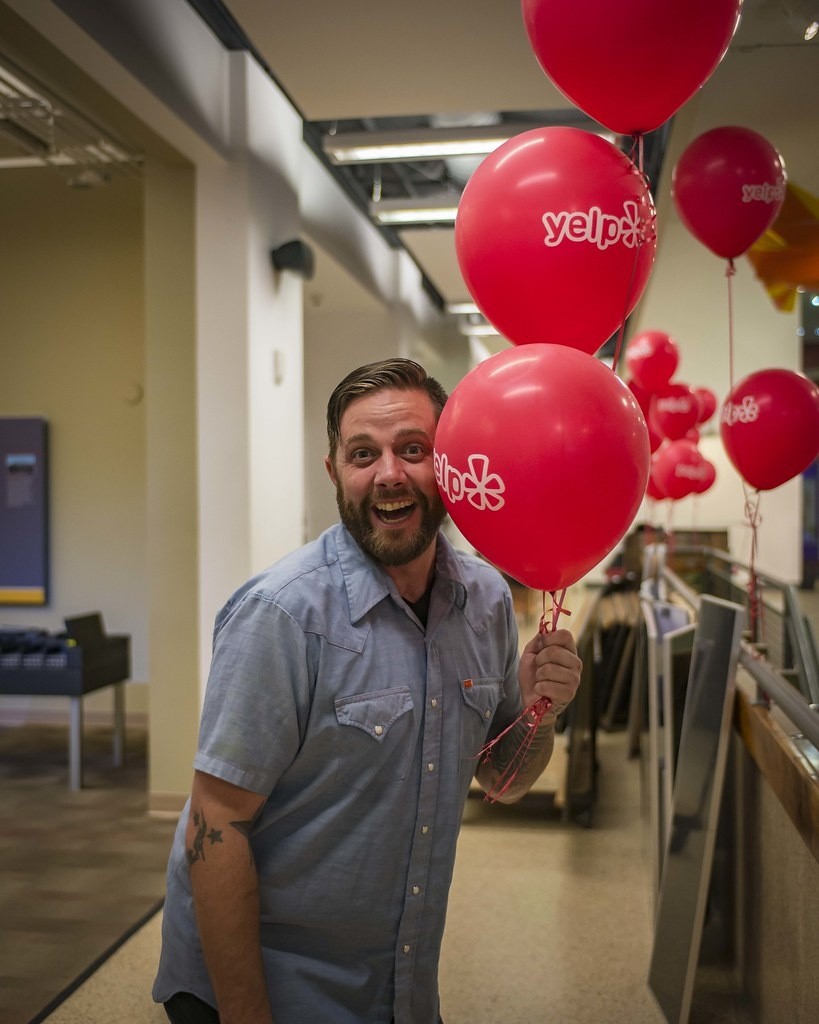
[673,127,786,259]
[622,379,716,502]
[434,341,653,596]
[717,370,818,494]
[624,330,677,388]
[518,4,741,136]
[454,128,660,356]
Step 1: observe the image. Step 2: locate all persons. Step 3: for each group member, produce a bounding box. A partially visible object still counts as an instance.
[148,356,583,1024]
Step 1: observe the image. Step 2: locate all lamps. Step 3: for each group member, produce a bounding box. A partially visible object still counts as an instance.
[459,324,501,337]
[365,191,466,229]
[321,121,623,168]
[444,300,481,318]
